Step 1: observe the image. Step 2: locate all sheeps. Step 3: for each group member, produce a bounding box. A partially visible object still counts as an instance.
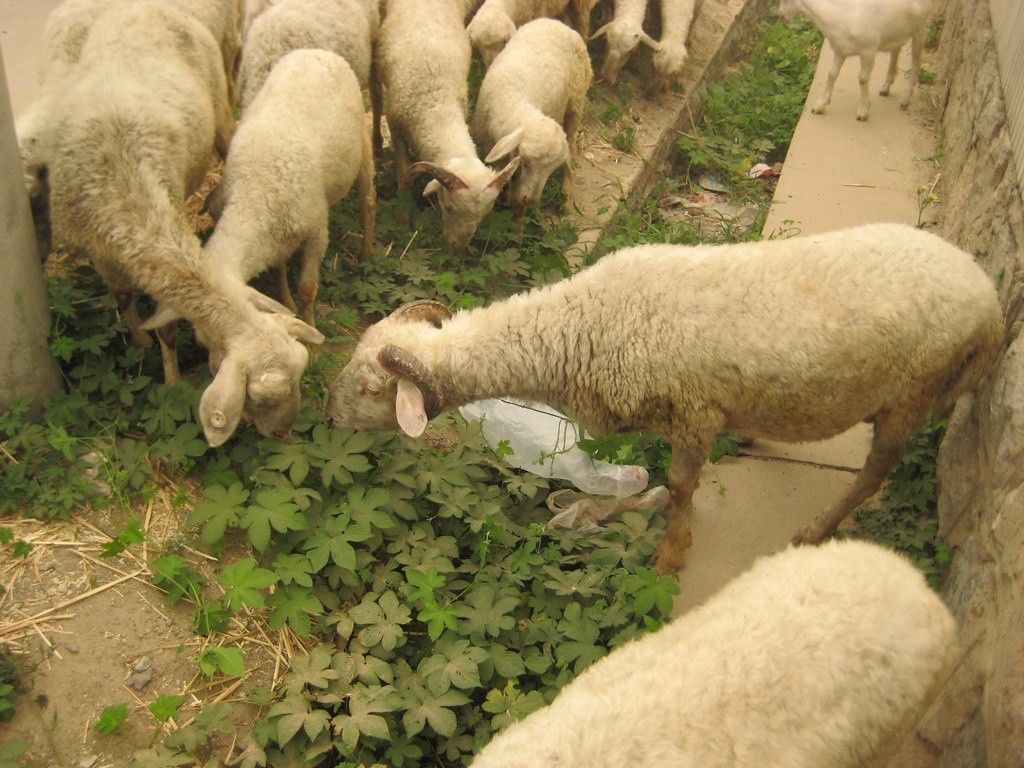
[778,0,935,123]
[464,537,963,767]
[325,223,1008,573]
[12,1,700,449]
